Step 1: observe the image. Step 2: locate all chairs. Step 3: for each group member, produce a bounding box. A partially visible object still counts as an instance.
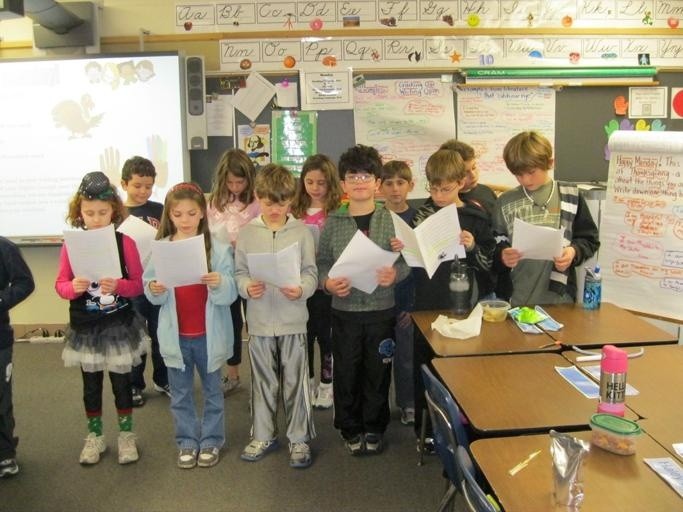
[421,364,471,511]
[455,446,497,512]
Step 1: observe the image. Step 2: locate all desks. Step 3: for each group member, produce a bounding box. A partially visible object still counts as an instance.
[636,420,683,463]
[515,303,680,350]
[410,309,562,439]
[431,352,641,437]
[469,429,683,512]
[562,345,683,419]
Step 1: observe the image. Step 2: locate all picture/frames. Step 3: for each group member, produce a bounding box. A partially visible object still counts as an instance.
[271,110,316,178]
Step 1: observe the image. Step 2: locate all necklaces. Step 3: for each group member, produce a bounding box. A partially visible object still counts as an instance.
[523,179,556,210]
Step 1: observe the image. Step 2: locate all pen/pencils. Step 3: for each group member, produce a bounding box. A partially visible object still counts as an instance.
[537,340,561,349]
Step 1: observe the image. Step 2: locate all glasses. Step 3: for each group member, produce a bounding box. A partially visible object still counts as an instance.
[425,179,461,194]
[340,174,378,185]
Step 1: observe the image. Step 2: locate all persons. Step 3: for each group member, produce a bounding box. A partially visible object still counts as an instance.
[235,163,319,467]
[439,140,498,216]
[317,145,412,455]
[492,131,600,305]
[55,173,143,465]
[142,184,238,469]
[206,150,258,398]
[380,160,416,425]
[293,153,343,410]
[387,148,496,456]
[117,156,171,407]
[0,236,35,477]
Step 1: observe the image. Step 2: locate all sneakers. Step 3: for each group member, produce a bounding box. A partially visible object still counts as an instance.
[197,444,223,469]
[307,377,317,407]
[218,374,245,399]
[130,386,146,408]
[239,438,280,462]
[415,435,438,457]
[79,432,107,466]
[175,442,201,471]
[287,440,314,469]
[116,430,141,465]
[0,456,21,479]
[150,378,173,398]
[344,432,364,457]
[399,404,418,428]
[363,431,385,455]
[316,379,334,411]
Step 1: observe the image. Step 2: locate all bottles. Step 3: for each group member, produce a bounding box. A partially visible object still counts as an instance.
[582,264,601,310]
[449,254,472,315]
[597,344,628,417]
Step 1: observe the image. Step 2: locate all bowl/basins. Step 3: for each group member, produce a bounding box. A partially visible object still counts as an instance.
[478,299,510,322]
[587,413,642,457]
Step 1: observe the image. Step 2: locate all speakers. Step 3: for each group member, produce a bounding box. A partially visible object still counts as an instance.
[184,55,209,152]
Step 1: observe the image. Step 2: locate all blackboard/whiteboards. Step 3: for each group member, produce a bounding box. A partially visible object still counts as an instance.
[188,65,683,208]
